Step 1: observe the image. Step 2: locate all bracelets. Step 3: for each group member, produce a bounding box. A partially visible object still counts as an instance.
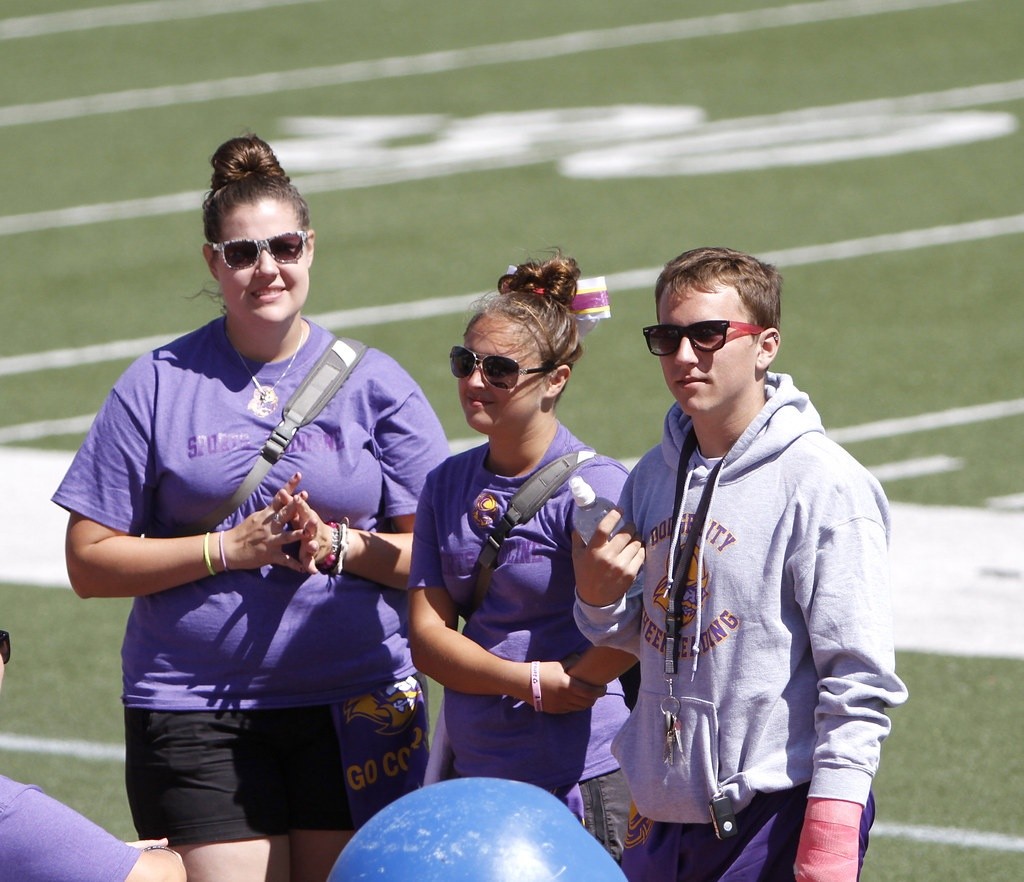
[319,521,348,575]
[204,532,216,576]
[531,660,544,712]
[219,530,228,572]
[142,845,182,860]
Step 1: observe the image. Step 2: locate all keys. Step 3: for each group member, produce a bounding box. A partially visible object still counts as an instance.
[661,711,683,765]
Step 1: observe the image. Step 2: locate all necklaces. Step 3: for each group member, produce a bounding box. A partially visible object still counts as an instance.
[225,319,304,412]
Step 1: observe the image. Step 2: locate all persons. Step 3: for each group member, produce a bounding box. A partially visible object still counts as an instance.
[0,630,187,882]
[569,246,908,882]
[407,252,631,863]
[63,137,452,882]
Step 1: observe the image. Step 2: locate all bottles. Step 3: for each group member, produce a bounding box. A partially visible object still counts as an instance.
[568,476,645,599]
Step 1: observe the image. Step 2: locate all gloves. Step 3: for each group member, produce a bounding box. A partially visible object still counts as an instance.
[794,796,864,881]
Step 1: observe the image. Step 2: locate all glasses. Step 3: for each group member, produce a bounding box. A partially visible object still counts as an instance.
[207,231,306,270]
[449,346,554,389]
[643,321,766,356]
[0,631,10,664]
